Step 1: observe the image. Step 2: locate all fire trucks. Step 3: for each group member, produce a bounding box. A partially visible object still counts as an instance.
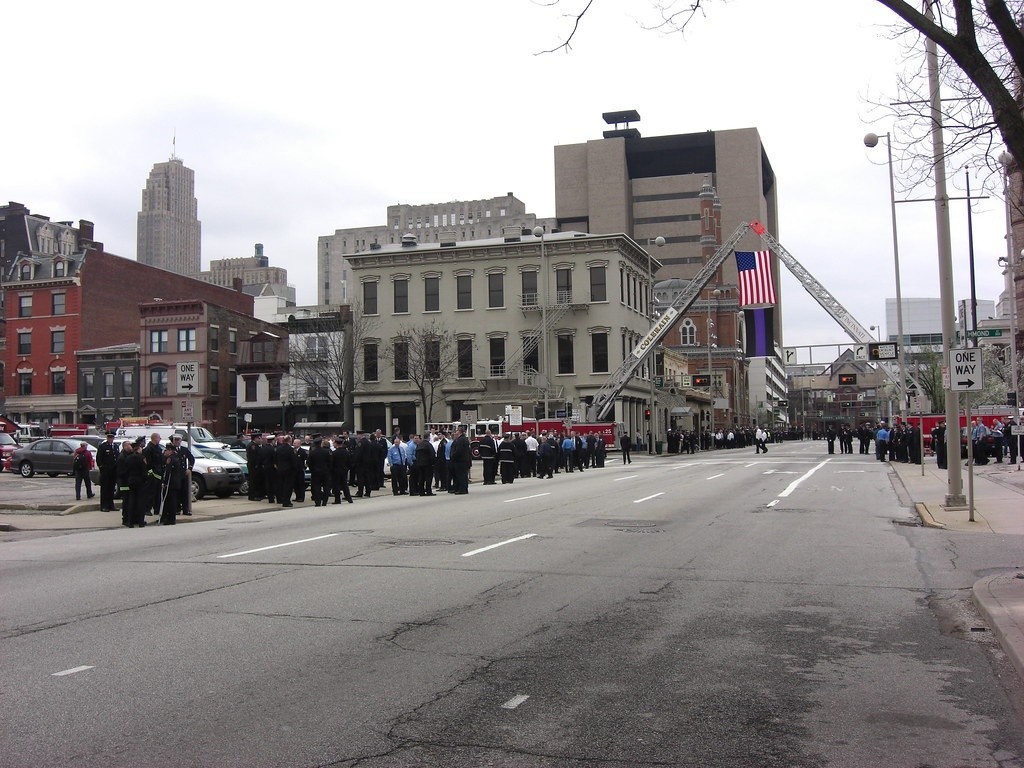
[750,218,1024,455]
[470,220,750,461]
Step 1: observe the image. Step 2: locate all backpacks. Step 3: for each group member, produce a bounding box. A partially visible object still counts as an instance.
[72,452,89,472]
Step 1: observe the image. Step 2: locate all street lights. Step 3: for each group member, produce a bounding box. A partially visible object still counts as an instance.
[869,324,885,420]
[864,131,907,428]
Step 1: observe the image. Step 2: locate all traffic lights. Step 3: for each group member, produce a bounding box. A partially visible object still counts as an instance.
[842,376,853,382]
[695,378,708,384]
[644,408,651,420]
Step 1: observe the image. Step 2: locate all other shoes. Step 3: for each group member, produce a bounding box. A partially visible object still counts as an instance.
[100,507,109,512]
[364,494,370,496]
[248,497,261,501]
[109,507,119,511]
[77,497,81,500]
[282,504,294,507]
[901,460,908,462]
[332,503,341,504]
[537,476,543,479]
[394,492,409,495]
[881,461,886,462]
[292,499,304,502]
[87,493,95,498]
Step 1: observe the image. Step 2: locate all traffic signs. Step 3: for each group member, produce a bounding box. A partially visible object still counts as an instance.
[948,347,984,391]
[175,360,200,395]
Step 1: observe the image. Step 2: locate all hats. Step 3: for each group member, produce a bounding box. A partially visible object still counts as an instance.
[136,436,145,442]
[1007,415,1014,418]
[356,431,365,433]
[880,420,885,423]
[105,431,116,435]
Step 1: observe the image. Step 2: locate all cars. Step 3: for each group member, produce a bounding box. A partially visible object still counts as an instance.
[0,415,404,503]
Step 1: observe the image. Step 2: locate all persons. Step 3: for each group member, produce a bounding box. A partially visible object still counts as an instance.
[713,426,804,449]
[115,433,195,528]
[931,420,947,469]
[755,425,762,453]
[620,431,632,464]
[960,412,1024,466]
[230,425,473,507]
[761,428,768,453]
[478,427,606,485]
[827,424,836,454]
[95,433,120,512]
[636,428,652,454]
[874,422,921,465]
[666,426,711,454]
[806,426,824,441]
[72,442,96,500]
[837,422,873,454]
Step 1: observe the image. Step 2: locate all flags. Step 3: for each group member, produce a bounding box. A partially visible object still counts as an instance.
[742,308,777,360]
[733,250,777,308]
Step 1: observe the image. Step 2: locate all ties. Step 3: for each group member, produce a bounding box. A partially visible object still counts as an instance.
[178,447,179,451]
[378,437,379,441]
[398,447,403,464]
